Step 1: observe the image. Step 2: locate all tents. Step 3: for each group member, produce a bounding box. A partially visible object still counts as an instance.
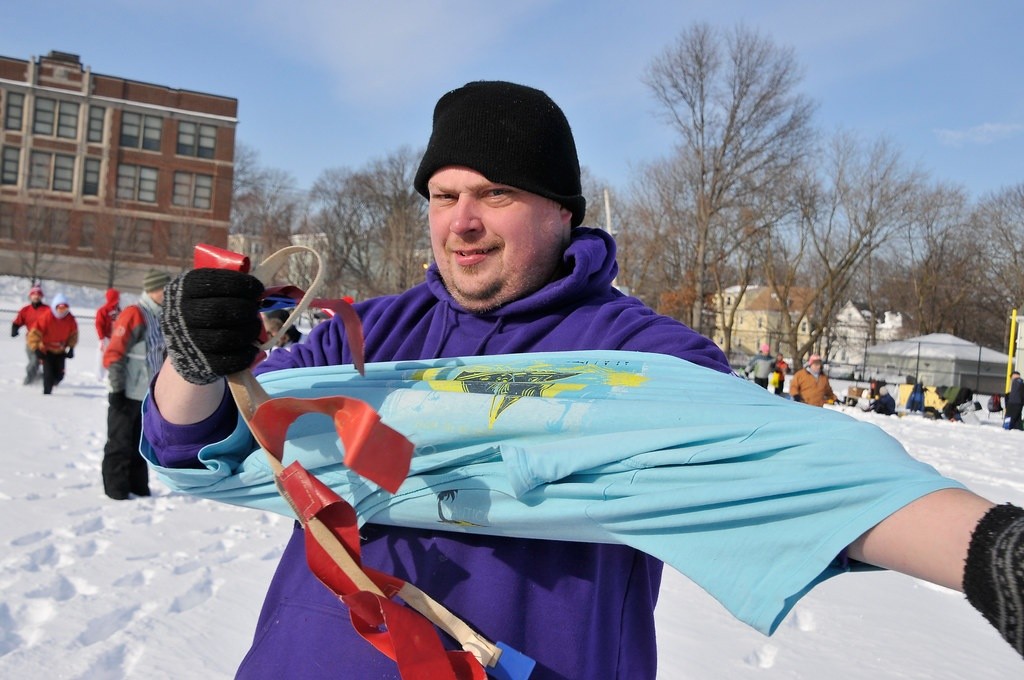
[862,332,1013,397]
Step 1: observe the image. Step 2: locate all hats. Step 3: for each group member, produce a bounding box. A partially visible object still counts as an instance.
[414,80,586,229]
[263,309,301,348]
[29,285,43,298]
[314,294,354,321]
[50,291,71,319]
[144,267,171,293]
[808,354,820,364]
[762,344,770,353]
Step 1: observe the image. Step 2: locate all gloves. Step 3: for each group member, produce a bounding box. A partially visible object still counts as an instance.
[157,268,263,386]
[35,349,46,361]
[67,347,72,358]
[962,503,1024,660]
[793,395,800,402]
[11,323,19,337]
[835,399,841,405]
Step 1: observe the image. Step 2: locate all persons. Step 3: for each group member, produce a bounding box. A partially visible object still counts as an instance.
[10,264,302,500]
[139,79,1024,680]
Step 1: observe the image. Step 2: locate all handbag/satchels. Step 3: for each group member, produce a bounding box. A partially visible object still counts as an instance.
[987,392,1003,412]
[922,401,963,423]
